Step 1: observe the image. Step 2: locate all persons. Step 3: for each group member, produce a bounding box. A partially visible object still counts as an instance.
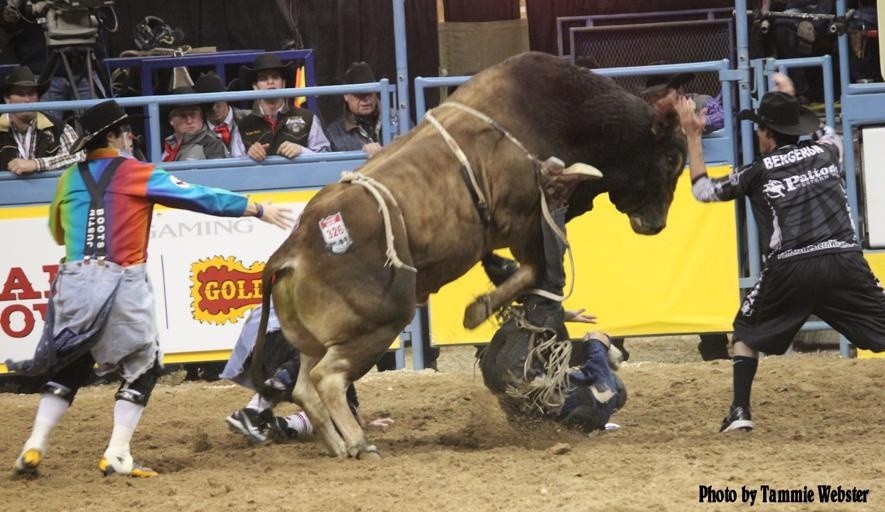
[218,298,394,448]
[483,156,629,438]
[229,53,332,161]
[160,86,230,161]
[195,71,252,159]
[675,71,884,431]
[0,64,88,176]
[9,100,296,477]
[3,0,116,123]
[323,60,415,153]
[638,60,725,135]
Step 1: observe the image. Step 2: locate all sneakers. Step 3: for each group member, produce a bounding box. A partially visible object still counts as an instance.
[718,404,755,433]
[99,452,158,478]
[13,449,42,474]
[226,406,297,444]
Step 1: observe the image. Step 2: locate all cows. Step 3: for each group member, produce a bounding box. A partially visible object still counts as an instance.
[250,52,697,459]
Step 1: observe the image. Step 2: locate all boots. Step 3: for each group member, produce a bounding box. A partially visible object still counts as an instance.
[542,155,603,209]
[482,252,523,304]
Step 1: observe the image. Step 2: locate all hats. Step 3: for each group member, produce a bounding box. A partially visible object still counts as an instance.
[168,54,296,108]
[70,100,149,155]
[0,66,51,104]
[342,62,377,83]
[738,91,820,136]
[637,61,694,96]
